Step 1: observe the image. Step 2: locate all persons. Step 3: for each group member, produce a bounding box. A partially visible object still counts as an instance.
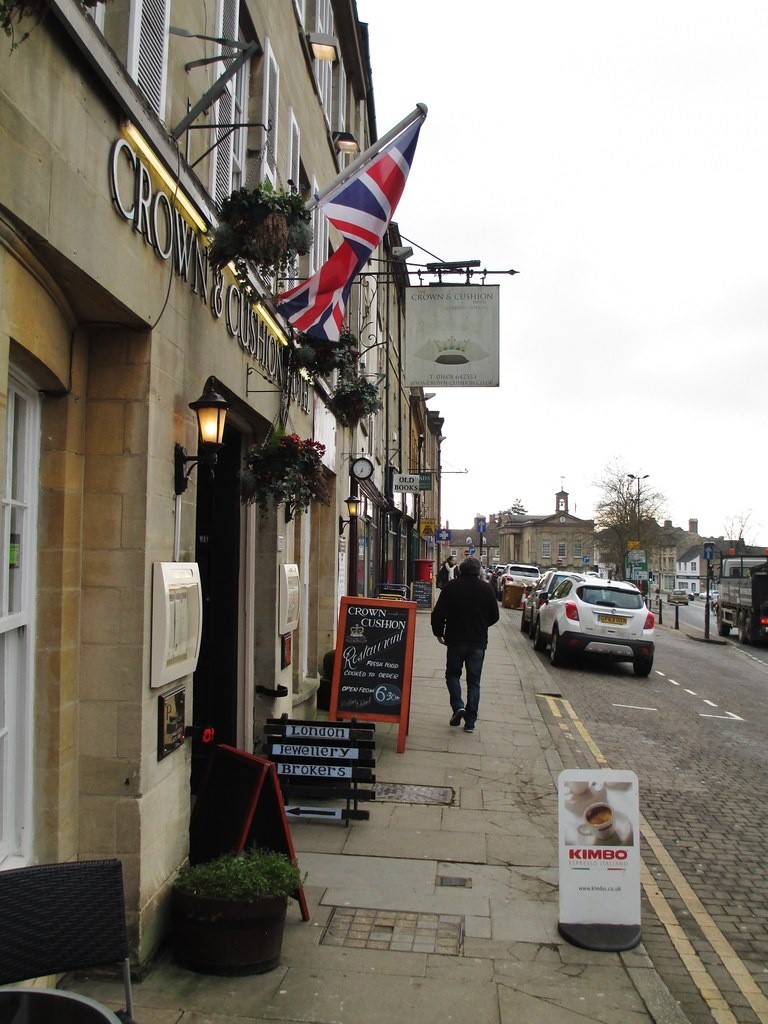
[459,551,486,582]
[431,556,500,733]
[435,556,460,591]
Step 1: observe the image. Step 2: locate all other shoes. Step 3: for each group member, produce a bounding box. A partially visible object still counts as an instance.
[450,707,466,726]
[464,723,476,733]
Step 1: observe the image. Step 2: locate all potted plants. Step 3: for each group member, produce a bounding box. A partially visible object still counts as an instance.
[292,314,389,428]
[168,847,308,974]
[205,180,316,271]
[243,431,329,520]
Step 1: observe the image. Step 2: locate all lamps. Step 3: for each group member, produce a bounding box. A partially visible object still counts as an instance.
[308,30,340,62]
[339,494,362,535]
[174,376,228,494]
[332,131,361,156]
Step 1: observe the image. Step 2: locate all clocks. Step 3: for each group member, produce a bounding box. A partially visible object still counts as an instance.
[352,453,374,479]
[559,516,567,524]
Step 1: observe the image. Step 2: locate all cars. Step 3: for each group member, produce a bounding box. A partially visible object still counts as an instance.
[687,591,696,601]
[700,589,719,616]
[670,589,689,604]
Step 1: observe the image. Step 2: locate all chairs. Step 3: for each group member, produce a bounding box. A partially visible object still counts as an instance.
[0,858,139,1024]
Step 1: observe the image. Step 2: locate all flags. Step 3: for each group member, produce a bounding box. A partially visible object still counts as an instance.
[274,117,427,344]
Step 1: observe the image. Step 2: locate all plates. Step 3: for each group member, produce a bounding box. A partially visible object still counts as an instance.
[578,812,631,846]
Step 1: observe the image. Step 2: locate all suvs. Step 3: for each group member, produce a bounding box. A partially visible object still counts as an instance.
[487,564,508,596]
[534,575,657,677]
[497,563,541,602]
[521,567,607,639]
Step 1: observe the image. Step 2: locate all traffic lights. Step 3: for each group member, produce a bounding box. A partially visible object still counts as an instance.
[649,570,653,584]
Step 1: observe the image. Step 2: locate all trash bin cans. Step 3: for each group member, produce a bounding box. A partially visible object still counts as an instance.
[502,577,527,609]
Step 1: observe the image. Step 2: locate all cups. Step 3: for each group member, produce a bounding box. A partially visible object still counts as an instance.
[577,803,614,840]
[563,781,631,795]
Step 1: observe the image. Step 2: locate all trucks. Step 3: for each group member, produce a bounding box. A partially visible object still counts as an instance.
[709,558,768,645]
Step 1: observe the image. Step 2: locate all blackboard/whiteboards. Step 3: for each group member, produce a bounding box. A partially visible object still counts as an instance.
[187,746,306,903]
[413,581,432,608]
[329,596,417,723]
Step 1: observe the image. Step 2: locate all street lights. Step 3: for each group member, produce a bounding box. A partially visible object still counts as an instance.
[627,473,649,596]
